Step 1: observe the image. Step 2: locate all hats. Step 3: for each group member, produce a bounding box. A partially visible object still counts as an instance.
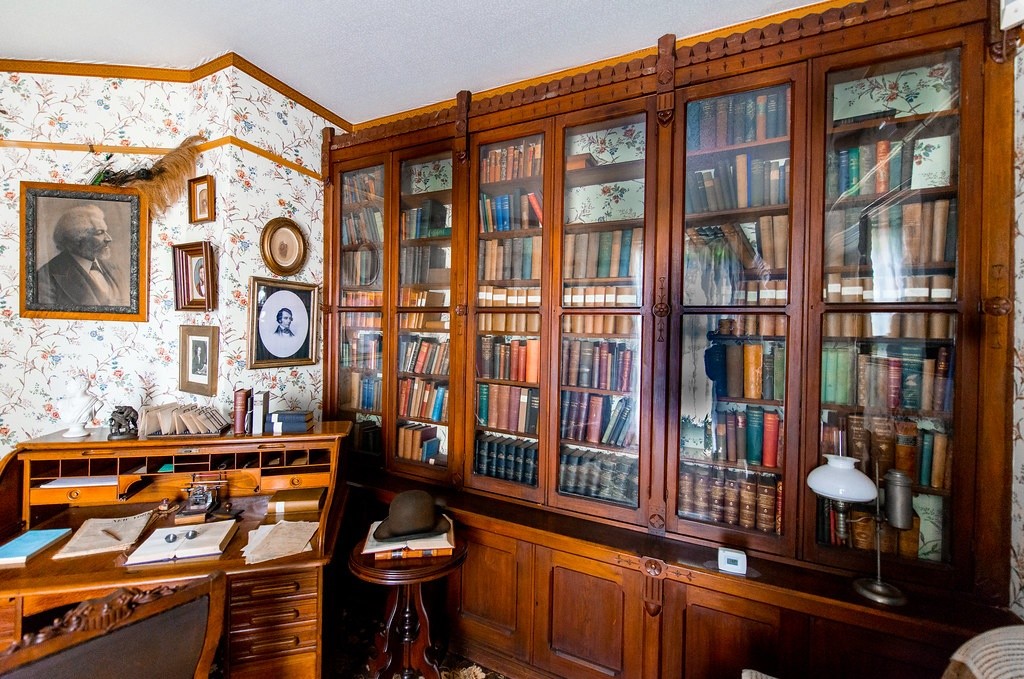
[373,491,451,542]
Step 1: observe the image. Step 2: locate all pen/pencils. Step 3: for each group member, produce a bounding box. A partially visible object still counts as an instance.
[102,530,120,541]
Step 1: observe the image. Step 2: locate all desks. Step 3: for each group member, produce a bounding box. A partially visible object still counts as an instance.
[348,535,468,679]
[0,421,356,679]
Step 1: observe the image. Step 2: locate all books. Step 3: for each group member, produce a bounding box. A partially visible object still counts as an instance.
[823,199,956,270]
[566,152,598,170]
[478,239,541,280]
[682,215,787,334]
[341,205,384,245]
[122,519,240,566]
[234,388,315,437]
[342,286,641,504]
[138,401,228,435]
[827,137,914,199]
[564,286,640,334]
[480,188,542,234]
[562,228,642,278]
[360,521,455,560]
[685,156,790,210]
[0,528,72,564]
[816,272,954,556]
[687,89,791,153]
[342,251,382,289]
[677,340,786,537]
[400,199,450,241]
[480,139,543,183]
[344,172,384,204]
[833,108,897,127]
[400,247,445,286]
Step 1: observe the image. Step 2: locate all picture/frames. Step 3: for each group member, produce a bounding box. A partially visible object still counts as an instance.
[187,175,215,224]
[171,240,215,313]
[178,325,219,397]
[20,181,149,322]
[259,218,308,276]
[347,239,380,285]
[246,276,319,370]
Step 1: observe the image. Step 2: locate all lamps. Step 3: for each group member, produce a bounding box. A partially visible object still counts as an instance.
[807,453,915,606]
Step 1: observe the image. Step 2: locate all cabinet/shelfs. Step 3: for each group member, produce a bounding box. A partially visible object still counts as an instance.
[326,0,1024,679]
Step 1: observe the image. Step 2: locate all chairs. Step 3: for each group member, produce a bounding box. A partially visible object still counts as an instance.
[0,570,226,678]
